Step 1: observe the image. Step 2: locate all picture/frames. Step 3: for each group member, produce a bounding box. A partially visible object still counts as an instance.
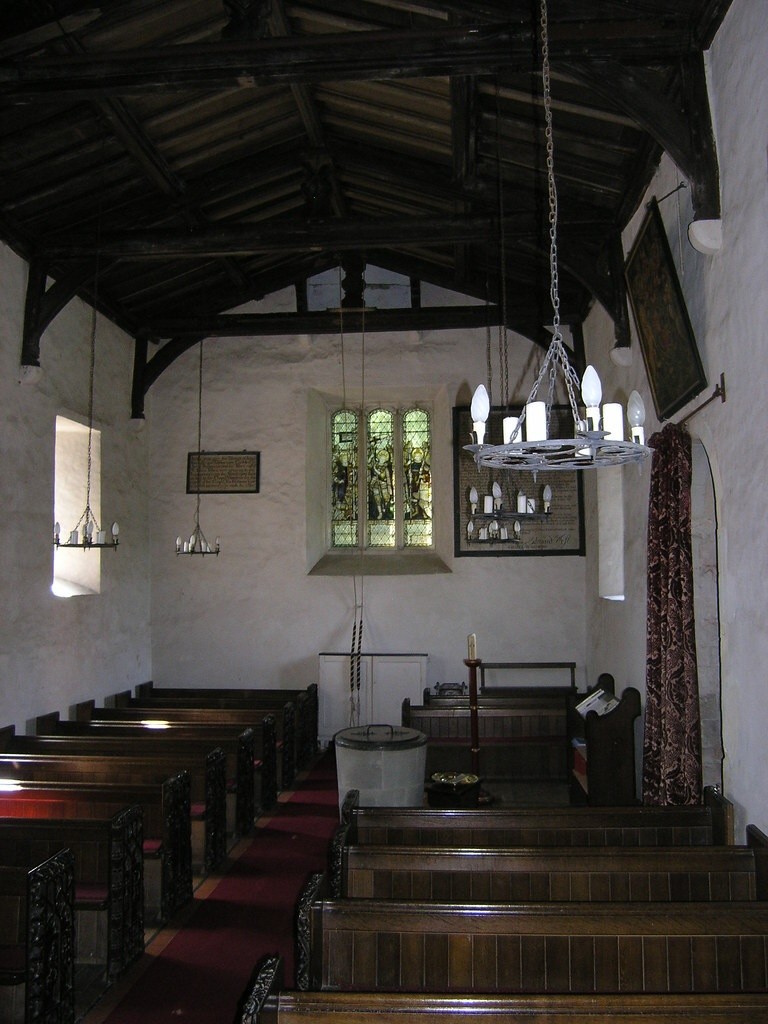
[620,195,709,423]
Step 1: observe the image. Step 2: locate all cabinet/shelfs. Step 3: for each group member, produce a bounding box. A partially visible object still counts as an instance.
[318,651,429,751]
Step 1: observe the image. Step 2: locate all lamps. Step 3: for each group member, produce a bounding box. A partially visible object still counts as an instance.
[51,257,222,558]
[460,0,656,548]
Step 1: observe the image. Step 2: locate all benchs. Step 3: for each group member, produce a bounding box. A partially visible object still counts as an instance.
[0,680,768,1024]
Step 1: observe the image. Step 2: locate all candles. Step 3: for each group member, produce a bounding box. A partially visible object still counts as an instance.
[468,634,476,661]
